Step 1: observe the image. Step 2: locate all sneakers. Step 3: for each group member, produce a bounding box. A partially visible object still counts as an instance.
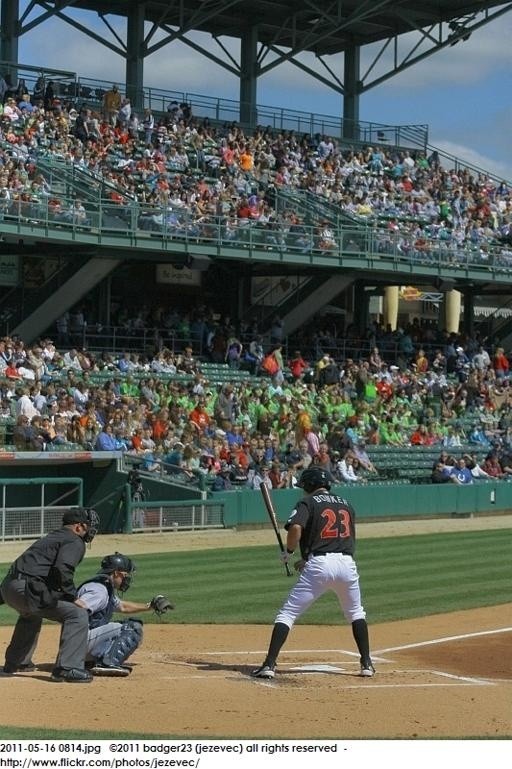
[250,664,275,678]
[360,663,375,677]
[90,660,133,676]
[4,660,39,673]
[51,665,93,683]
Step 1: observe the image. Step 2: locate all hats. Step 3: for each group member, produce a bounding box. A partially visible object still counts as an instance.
[41,384,511,451]
[62,507,91,526]
[320,346,511,380]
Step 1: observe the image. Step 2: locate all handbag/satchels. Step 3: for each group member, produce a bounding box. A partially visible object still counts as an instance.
[262,352,277,375]
[246,351,256,363]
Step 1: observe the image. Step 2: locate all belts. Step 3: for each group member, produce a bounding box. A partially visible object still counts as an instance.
[8,570,28,579]
[313,551,351,557]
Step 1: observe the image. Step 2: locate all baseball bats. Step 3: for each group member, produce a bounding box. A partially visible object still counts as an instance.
[261,482,291,577]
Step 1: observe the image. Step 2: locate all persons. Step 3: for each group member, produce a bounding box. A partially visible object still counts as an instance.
[0,298,512,488]
[77,552,174,675]
[249,471,376,677]
[0,74,512,260]
[0,504,101,683]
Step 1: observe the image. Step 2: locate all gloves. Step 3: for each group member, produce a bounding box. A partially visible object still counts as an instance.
[294,560,306,571]
[280,549,293,561]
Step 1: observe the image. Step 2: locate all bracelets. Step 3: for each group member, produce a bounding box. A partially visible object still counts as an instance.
[287,549,294,554]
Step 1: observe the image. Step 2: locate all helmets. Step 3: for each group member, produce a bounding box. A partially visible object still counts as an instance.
[293,467,333,494]
[96,552,133,575]
